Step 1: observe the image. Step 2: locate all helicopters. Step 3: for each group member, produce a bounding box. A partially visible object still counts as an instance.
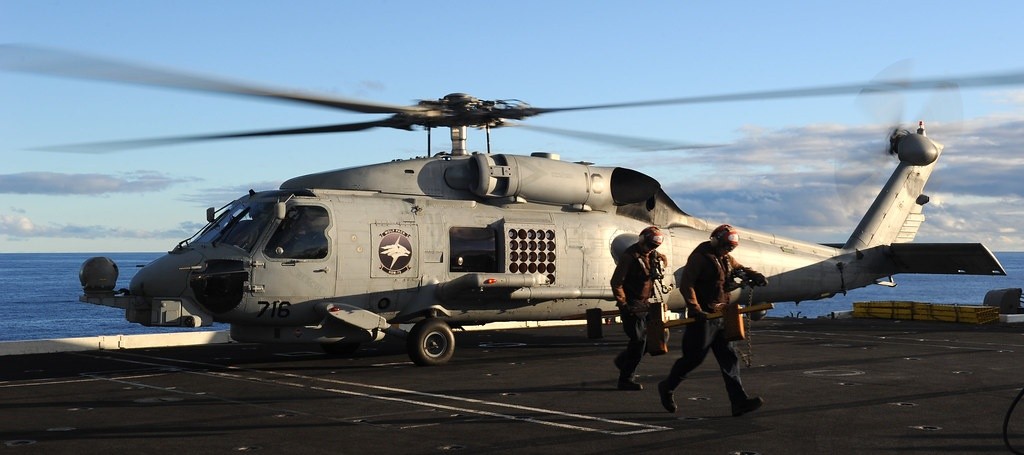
[0,46,1024,368]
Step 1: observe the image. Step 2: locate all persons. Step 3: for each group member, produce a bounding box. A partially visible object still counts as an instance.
[658,224,763,416]
[611,226,669,391]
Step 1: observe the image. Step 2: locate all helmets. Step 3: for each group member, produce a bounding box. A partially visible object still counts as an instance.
[709,224,739,250]
[638,226,664,248]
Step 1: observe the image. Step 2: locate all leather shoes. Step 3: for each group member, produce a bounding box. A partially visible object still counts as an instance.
[617,377,643,391]
[613,353,623,369]
[729,396,764,417]
[658,381,678,413]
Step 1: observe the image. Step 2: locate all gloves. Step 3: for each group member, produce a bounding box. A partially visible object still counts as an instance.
[746,271,769,287]
[618,303,631,316]
[695,311,709,324]
